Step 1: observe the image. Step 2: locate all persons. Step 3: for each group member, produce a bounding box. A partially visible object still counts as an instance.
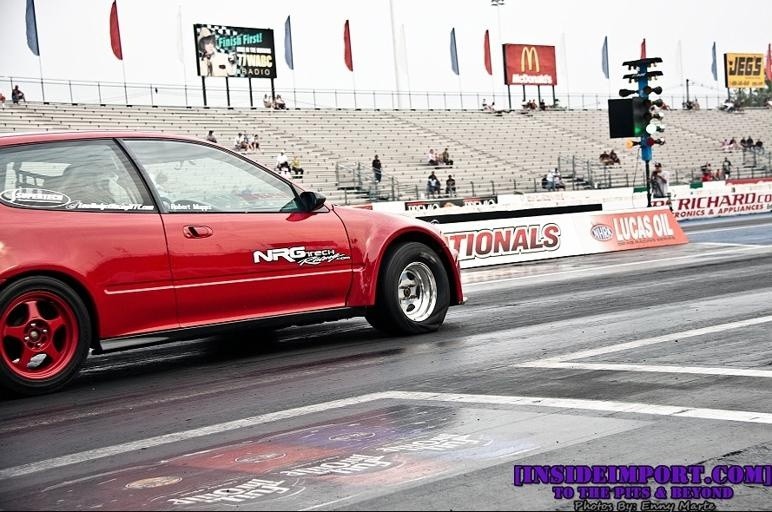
[701,157,731,181]
[521,97,547,111]
[197,28,237,76]
[428,171,442,199]
[264,94,286,110]
[682,98,699,110]
[542,168,561,192]
[156,171,170,203]
[720,136,763,153]
[445,175,456,198]
[234,132,259,155]
[371,155,381,181]
[13,84,27,103]
[651,163,669,198]
[0,93,6,106]
[600,149,622,168]
[723,99,743,112]
[484,102,496,111]
[207,129,217,143]
[276,150,304,179]
[428,147,453,169]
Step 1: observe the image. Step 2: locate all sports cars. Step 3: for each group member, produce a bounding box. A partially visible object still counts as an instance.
[0,133,468,394]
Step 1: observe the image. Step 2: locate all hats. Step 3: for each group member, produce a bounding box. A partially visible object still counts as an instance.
[655,163,661,167]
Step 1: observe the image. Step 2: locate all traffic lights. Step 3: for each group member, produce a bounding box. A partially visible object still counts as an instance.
[608,97,652,138]
